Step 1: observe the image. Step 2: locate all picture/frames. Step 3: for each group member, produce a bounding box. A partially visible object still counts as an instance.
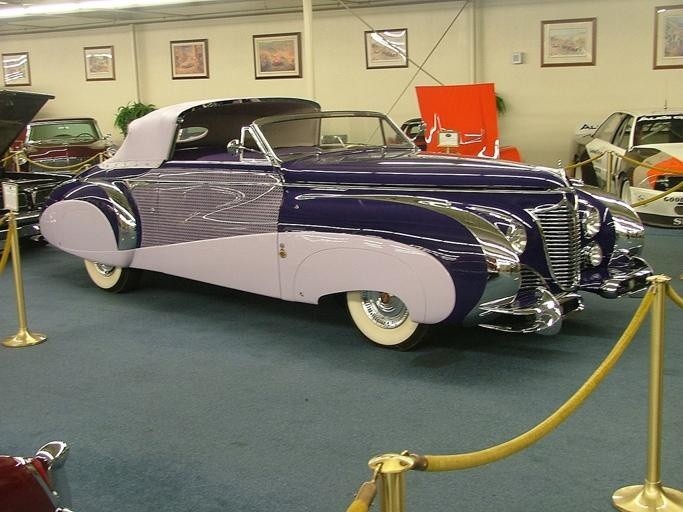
[365,28,408,70]
[540,17,597,67]
[653,4,683,70]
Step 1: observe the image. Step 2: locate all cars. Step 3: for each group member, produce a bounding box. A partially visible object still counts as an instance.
[571,109,683,229]
[0,89,118,249]
[9,117,114,179]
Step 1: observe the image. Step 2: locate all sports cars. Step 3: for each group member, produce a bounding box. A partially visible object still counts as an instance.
[39,96,654,351]
